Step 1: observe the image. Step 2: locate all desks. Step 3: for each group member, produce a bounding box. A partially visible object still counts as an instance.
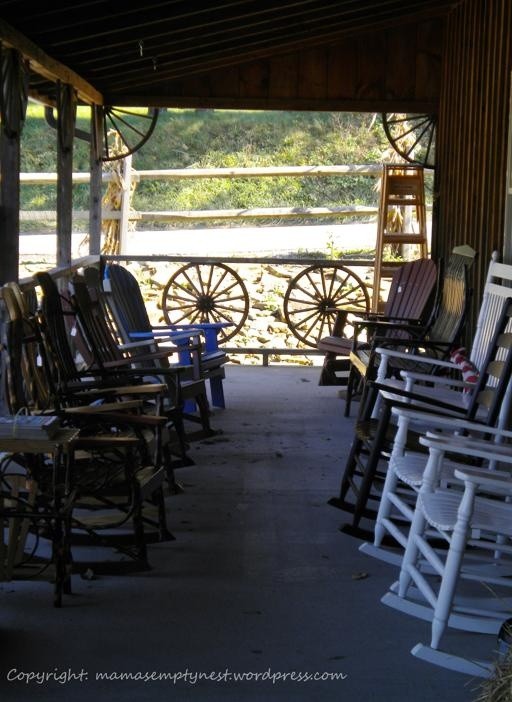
[0,424,80,608]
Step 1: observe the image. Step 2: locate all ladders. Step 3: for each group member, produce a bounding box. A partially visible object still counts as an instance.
[372,163,428,315]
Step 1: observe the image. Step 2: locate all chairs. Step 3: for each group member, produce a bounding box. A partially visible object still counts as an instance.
[317,251,512,679]
[0,263,234,570]
